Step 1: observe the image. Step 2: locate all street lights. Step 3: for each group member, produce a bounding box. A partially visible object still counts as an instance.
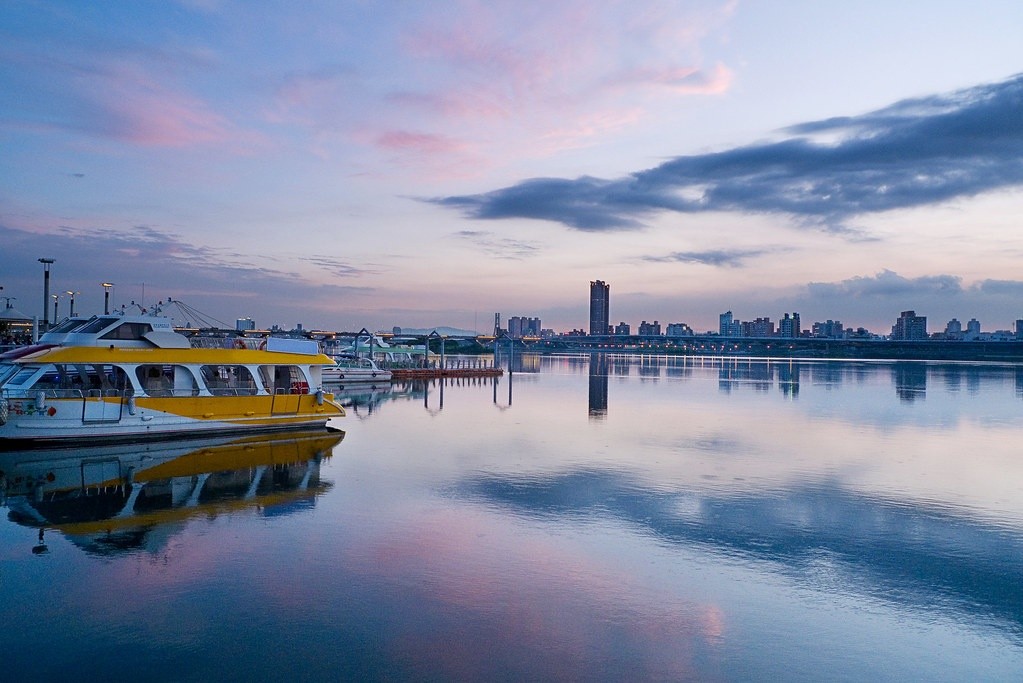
[38,257,56,332]
[99,283,114,314]
[51,294,64,324]
[64,290,80,317]
[1,296,16,309]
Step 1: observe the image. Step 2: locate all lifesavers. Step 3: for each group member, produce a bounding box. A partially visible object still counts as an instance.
[340,385,344,390]
[232,339,247,349]
[372,373,376,377]
[340,374,344,379]
[418,357,425,365]
[372,385,376,389]
[258,340,267,350]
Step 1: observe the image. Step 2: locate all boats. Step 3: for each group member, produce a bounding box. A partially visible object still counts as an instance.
[322,358,393,382]
[0,314,347,443]
[322,383,393,405]
[0,427,346,558]
